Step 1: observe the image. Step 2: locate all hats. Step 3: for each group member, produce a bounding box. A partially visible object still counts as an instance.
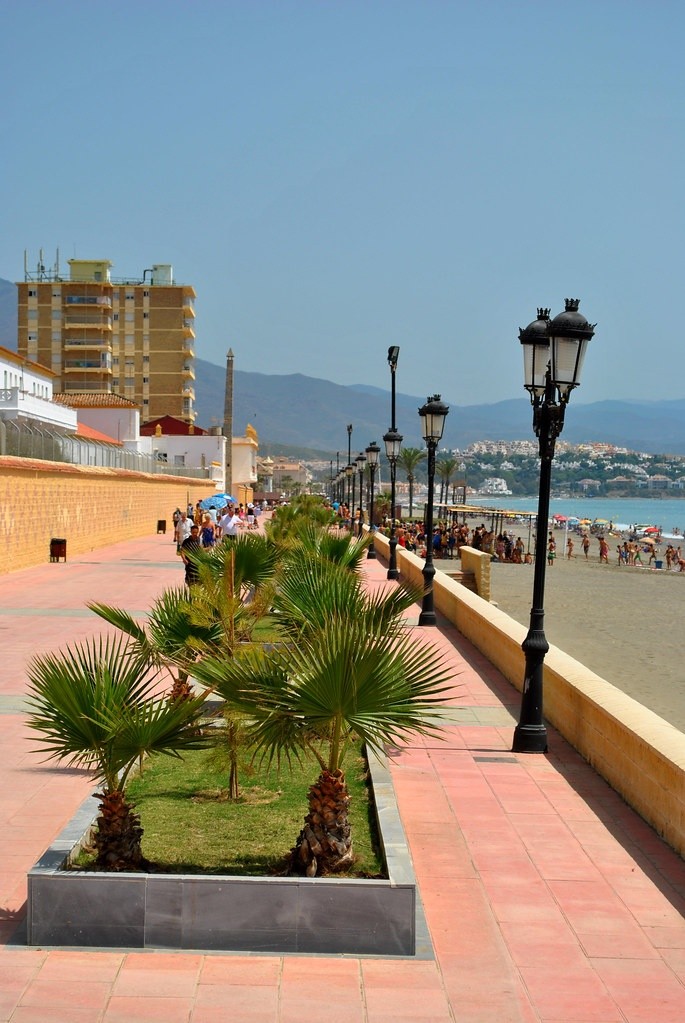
[248,503,254,508]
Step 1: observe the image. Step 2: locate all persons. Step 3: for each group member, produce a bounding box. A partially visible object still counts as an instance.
[172,499,259,592]
[369,498,537,565]
[252,497,361,531]
[548,516,685,573]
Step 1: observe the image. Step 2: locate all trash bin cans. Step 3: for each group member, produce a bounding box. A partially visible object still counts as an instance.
[157,520,166,534]
[654,560,663,568]
[51,538,66,562]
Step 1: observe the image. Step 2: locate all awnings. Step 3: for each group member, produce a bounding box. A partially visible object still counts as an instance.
[639,536,656,545]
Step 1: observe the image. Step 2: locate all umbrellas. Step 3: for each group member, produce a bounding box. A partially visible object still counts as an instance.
[644,528,659,532]
[502,510,609,530]
[213,493,237,503]
[200,498,228,510]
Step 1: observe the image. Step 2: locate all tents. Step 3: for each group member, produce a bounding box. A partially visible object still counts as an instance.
[403,503,418,509]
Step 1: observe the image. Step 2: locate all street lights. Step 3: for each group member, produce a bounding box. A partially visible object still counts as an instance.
[418,393,450,626]
[383,346,404,581]
[328,423,382,559]
[511,298,598,755]
